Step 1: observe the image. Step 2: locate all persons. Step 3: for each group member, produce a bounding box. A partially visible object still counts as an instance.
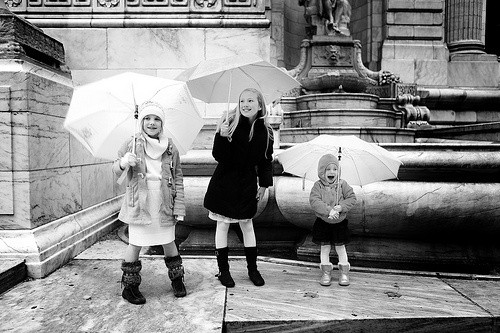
[202,87,272,288]
[113,104,187,305]
[310,152,358,286]
[308,0,352,31]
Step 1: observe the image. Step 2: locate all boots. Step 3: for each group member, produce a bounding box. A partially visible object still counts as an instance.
[120,259,147,305]
[244,247,265,286]
[338,262,351,286]
[215,245,236,288]
[318,262,334,286]
[164,253,187,298]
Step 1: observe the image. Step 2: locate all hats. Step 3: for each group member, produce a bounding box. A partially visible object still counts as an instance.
[137,99,164,128]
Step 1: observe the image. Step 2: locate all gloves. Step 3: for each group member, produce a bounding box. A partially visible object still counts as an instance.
[120,151,139,170]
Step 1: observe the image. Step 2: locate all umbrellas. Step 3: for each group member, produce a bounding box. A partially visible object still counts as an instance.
[275,135,405,205]
[186,52,303,123]
[63,71,205,168]
